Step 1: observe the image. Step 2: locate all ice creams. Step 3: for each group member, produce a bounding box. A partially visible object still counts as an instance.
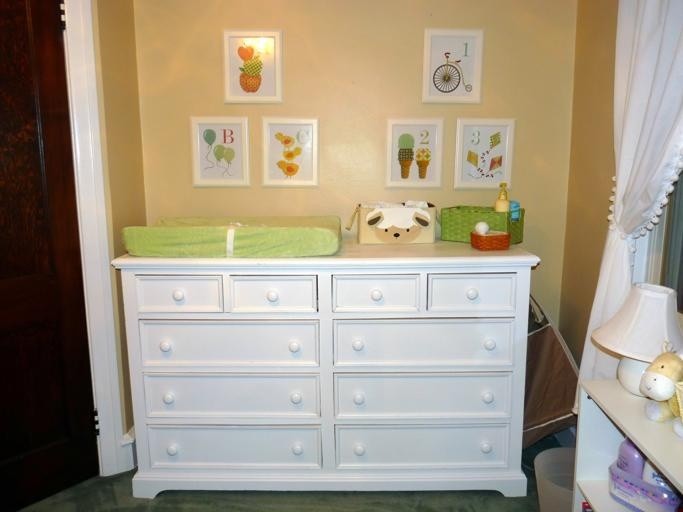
[398,133,415,179]
[415,147,432,179]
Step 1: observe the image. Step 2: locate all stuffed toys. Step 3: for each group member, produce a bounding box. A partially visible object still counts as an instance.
[640,341,683,439]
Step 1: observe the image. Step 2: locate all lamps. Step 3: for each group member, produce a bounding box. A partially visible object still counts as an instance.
[588,279,681,398]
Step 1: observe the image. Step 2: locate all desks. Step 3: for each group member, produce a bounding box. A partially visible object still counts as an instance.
[567,375,682,512]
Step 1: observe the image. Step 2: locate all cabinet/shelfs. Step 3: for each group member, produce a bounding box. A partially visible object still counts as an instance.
[112,248,329,501]
[327,245,542,501]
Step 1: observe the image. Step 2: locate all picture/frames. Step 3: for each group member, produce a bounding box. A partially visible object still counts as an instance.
[262,118,319,187]
[384,118,443,188]
[453,117,514,191]
[189,115,249,187]
[222,28,283,103]
[422,27,483,105]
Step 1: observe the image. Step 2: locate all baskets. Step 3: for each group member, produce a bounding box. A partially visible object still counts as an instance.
[438,205,526,247]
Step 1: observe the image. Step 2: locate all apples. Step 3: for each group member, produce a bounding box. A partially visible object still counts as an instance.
[239,73,262,93]
[238,45,254,60]
